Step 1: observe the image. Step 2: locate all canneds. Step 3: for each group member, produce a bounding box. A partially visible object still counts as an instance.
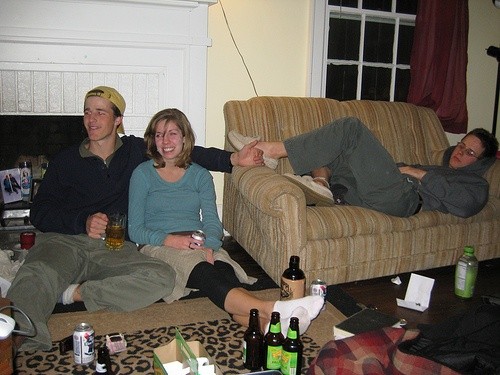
[309,279,327,311]
[192,230,206,246]
[72,323,95,364]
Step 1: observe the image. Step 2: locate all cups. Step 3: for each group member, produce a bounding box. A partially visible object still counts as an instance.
[99,212,127,251]
[20,231,35,249]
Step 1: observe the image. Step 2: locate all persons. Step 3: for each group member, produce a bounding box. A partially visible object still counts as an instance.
[127,109,324,335]
[228,116,499,219]
[0,86,176,350]
[4,174,21,194]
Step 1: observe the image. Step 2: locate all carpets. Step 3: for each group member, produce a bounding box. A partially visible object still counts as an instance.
[12,288,348,375]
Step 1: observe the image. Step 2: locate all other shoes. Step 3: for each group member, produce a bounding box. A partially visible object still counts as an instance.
[282,172,335,208]
[227,130,279,170]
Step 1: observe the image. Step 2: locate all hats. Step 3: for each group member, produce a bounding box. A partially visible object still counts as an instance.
[84,85,126,134]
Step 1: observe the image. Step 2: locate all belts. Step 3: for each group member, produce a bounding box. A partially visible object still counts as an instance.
[412,192,423,217]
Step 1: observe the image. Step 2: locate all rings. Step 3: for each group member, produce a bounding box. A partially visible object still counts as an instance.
[189,243,191,248]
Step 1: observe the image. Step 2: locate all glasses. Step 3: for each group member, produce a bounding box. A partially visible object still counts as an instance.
[456,141,480,161]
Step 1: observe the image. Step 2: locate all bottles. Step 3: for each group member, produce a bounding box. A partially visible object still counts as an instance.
[262,312,284,372]
[40,162,50,178]
[19,161,33,201]
[241,308,264,369]
[281,316,303,375]
[280,255,304,301]
[95,348,113,375]
[455,246,477,297]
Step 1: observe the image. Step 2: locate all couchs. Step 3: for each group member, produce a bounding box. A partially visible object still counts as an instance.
[222,96,500,296]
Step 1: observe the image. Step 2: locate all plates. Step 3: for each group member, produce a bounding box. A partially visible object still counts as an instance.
[11,243,35,251]
